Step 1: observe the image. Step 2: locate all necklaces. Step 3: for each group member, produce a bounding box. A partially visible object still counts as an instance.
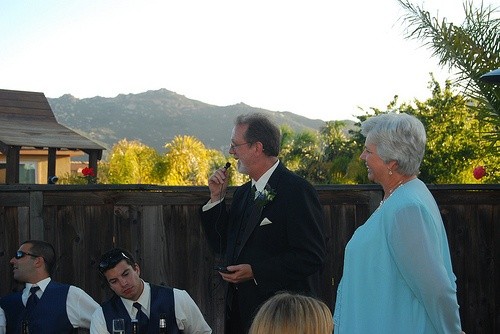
[379,177,407,205]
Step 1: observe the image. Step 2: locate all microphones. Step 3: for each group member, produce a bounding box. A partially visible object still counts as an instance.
[224,162,231,170]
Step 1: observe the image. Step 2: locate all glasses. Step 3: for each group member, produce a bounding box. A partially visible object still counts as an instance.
[98,252,129,268]
[230,141,254,152]
[15,250,38,259]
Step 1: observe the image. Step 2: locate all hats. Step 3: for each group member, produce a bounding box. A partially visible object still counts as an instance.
[479,68,500,83]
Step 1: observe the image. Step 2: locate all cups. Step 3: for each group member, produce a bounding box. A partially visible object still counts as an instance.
[112,318,125,334]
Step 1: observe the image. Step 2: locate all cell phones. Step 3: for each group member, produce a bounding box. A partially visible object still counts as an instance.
[217,266,236,274]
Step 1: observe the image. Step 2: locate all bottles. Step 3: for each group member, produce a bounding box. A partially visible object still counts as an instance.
[159,313,167,334]
[131,319,138,334]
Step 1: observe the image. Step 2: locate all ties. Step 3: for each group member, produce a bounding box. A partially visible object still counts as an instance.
[133,301,149,334]
[25,286,40,313]
[246,185,257,211]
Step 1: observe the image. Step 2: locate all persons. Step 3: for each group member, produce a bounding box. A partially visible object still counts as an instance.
[0,240,100,334]
[248,293,333,334]
[201,113,325,334]
[334,114,465,334]
[90,249,212,334]
